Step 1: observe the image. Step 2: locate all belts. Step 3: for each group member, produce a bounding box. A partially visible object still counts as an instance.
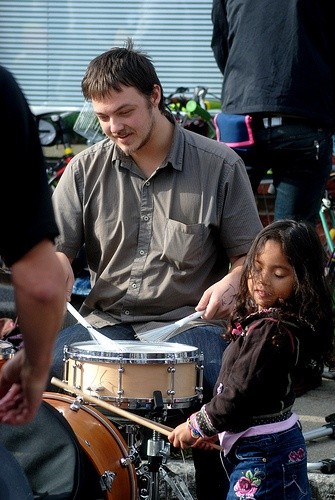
[255,115,330,131]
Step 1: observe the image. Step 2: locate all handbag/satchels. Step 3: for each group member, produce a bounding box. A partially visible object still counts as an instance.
[214,113,254,152]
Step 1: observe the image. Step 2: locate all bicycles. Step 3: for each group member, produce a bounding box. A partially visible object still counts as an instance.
[35,85,219,192]
[295,179,335,397]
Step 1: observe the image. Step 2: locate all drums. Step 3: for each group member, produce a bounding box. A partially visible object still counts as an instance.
[0,390,139,500]
[61,340,206,410]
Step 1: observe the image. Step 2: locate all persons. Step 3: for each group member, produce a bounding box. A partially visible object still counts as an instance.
[45,38,264,500]
[211,1,335,398]
[0,66,68,500]
[168,219,326,500]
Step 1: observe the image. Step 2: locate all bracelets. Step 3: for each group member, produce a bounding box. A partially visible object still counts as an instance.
[187,417,201,439]
